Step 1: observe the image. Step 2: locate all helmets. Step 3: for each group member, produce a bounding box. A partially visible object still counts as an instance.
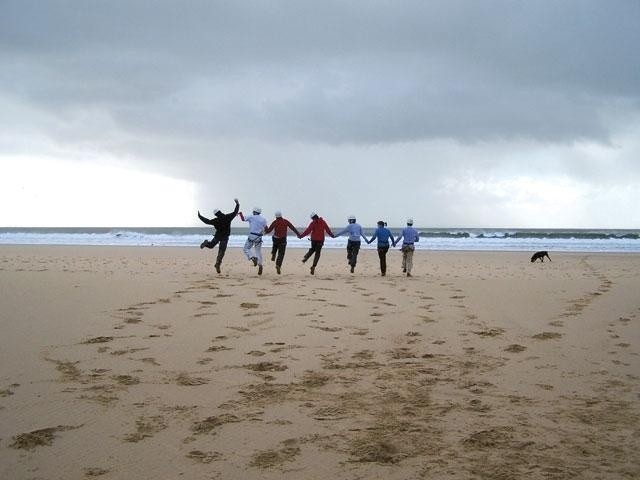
[213,207,414,225]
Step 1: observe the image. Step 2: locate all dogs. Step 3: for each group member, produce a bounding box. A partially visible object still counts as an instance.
[530,251,552,263]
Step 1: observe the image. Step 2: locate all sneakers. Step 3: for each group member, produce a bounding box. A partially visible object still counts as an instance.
[200,240,412,276]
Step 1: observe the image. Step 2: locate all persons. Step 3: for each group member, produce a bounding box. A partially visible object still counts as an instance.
[391,218,419,276]
[333,215,369,273]
[198,198,240,274]
[265,211,300,274]
[300,210,334,275]
[238,207,268,275]
[367,221,396,276]
